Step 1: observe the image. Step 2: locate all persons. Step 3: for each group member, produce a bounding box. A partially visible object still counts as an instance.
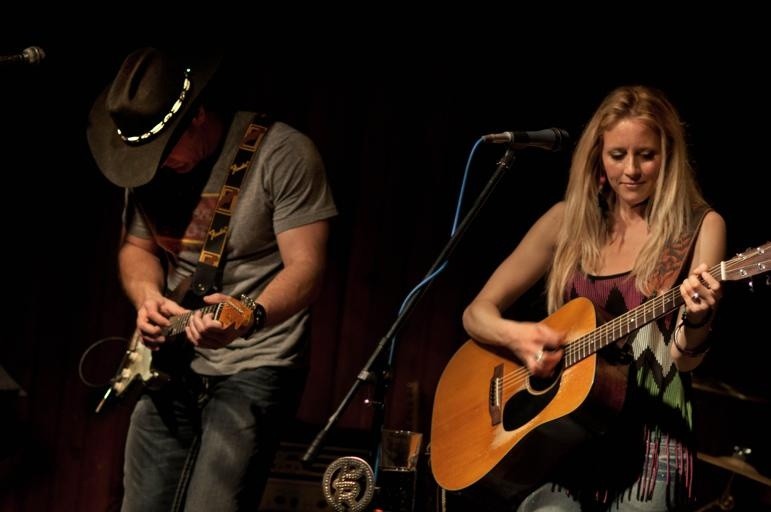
[86,48,337,512]
[461,87,726,512]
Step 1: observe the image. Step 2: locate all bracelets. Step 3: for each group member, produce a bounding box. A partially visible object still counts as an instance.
[679,311,711,329]
[247,302,268,338]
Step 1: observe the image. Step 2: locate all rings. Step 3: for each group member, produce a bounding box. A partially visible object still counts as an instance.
[691,293,701,302]
[533,350,545,362]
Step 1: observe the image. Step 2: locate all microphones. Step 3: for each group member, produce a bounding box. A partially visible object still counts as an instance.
[0,45,46,66]
[481,127,570,152]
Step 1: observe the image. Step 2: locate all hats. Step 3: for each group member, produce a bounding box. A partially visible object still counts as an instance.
[87,51,218,188]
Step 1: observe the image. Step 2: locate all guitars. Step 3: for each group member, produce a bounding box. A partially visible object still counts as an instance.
[109,293,265,399]
[428,239,771,502]
